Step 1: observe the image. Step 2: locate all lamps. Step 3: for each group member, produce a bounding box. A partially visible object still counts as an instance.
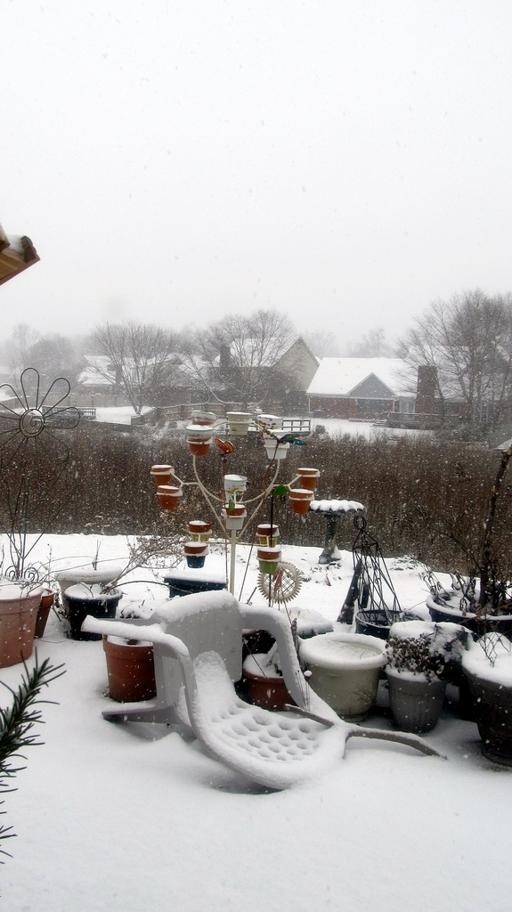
[148,409,320,599]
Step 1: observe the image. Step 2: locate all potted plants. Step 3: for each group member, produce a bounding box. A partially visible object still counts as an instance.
[459,610,512,769]
[424,450,512,635]
[382,625,455,734]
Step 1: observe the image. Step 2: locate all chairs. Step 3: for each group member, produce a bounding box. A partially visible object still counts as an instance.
[82,585,448,791]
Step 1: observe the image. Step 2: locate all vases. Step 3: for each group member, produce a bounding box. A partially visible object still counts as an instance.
[35,589,57,639]
[64,583,123,642]
[58,565,121,597]
[1,582,43,666]
[162,573,226,598]
[243,656,295,711]
[297,625,334,681]
[101,632,156,705]
[300,632,387,723]
[354,608,424,638]
[240,628,275,660]
